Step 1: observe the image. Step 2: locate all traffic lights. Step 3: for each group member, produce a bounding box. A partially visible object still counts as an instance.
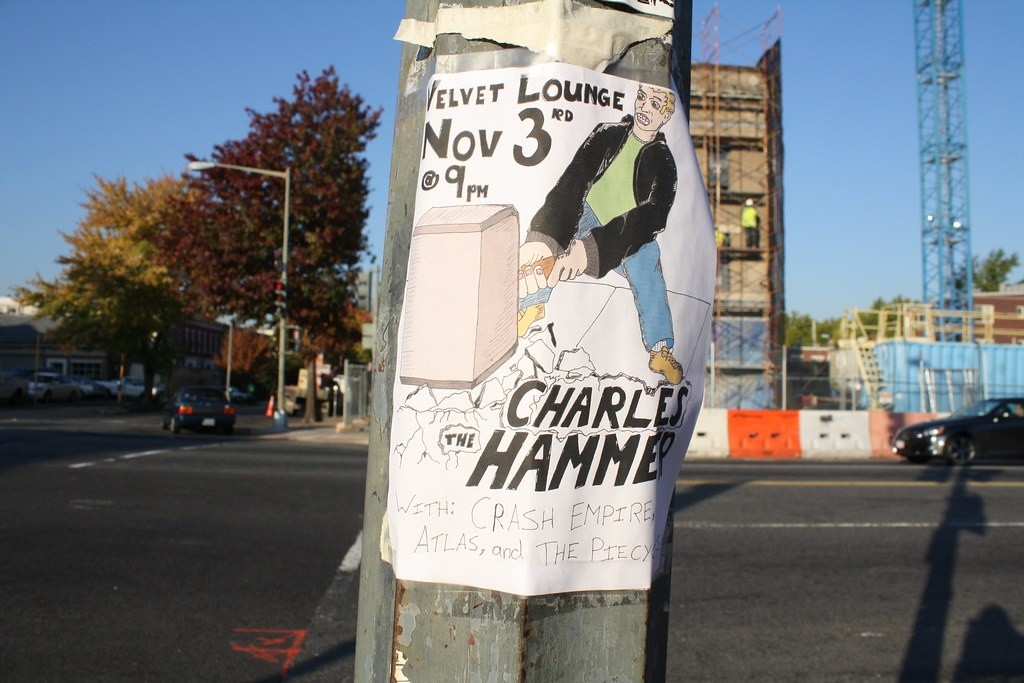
[273,281,285,321]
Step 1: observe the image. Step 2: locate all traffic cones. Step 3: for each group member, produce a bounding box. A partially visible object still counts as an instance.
[265,394,274,417]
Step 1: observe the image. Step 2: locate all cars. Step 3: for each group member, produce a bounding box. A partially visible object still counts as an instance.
[0,368,164,405]
[162,387,236,434]
[891,397,1024,464]
[229,387,256,404]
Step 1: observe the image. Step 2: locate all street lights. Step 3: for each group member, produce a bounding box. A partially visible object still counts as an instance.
[189,162,288,431]
